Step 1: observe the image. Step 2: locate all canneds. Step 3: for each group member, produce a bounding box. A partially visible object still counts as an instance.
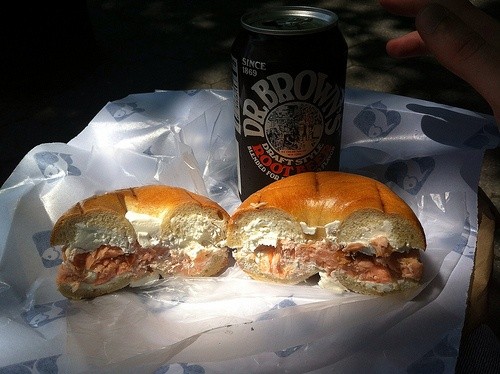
[233,5,347,199]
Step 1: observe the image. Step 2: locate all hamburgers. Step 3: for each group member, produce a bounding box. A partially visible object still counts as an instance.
[226,170,427,295]
[49,185,231,299]
